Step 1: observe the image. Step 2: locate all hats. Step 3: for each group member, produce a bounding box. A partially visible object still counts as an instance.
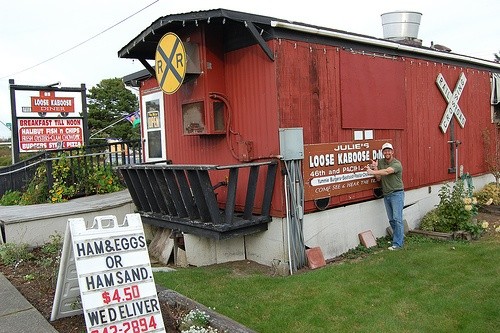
[382,143,393,151]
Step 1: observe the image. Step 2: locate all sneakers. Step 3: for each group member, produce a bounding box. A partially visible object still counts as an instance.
[388,246,396,250]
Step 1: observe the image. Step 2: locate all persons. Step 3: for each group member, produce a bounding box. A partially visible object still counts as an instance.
[368,143,405,252]
